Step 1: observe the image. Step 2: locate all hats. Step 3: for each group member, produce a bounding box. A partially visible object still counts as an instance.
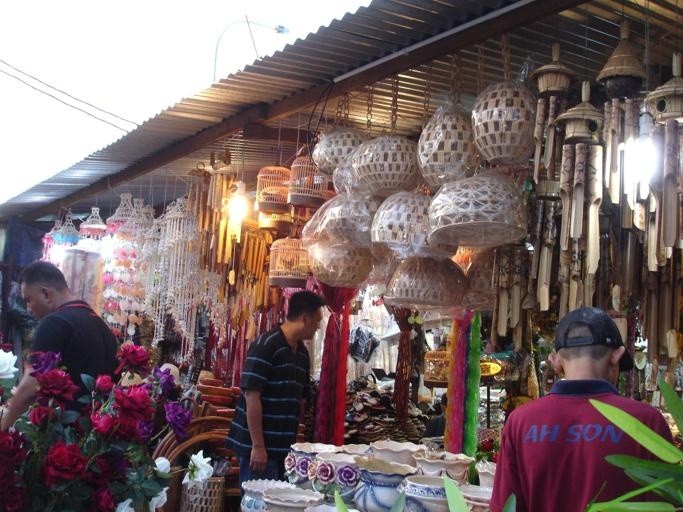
[556,307,634,371]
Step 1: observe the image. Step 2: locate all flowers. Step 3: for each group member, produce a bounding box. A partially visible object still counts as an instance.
[0,329,215,512]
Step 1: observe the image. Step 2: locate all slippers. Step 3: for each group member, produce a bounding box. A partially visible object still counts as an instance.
[344,374,430,444]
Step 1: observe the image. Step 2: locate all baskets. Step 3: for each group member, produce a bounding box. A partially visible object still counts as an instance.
[180,477,225,512]
[308,81,538,312]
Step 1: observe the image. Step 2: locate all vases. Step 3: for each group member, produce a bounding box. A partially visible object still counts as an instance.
[239,440,497,512]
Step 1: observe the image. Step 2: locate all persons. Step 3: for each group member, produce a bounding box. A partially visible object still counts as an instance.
[0,259,125,434]
[220,288,327,498]
[487,303,676,509]
[422,391,448,438]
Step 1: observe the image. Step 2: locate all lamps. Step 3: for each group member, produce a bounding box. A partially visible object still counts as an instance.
[227,124,250,221]
